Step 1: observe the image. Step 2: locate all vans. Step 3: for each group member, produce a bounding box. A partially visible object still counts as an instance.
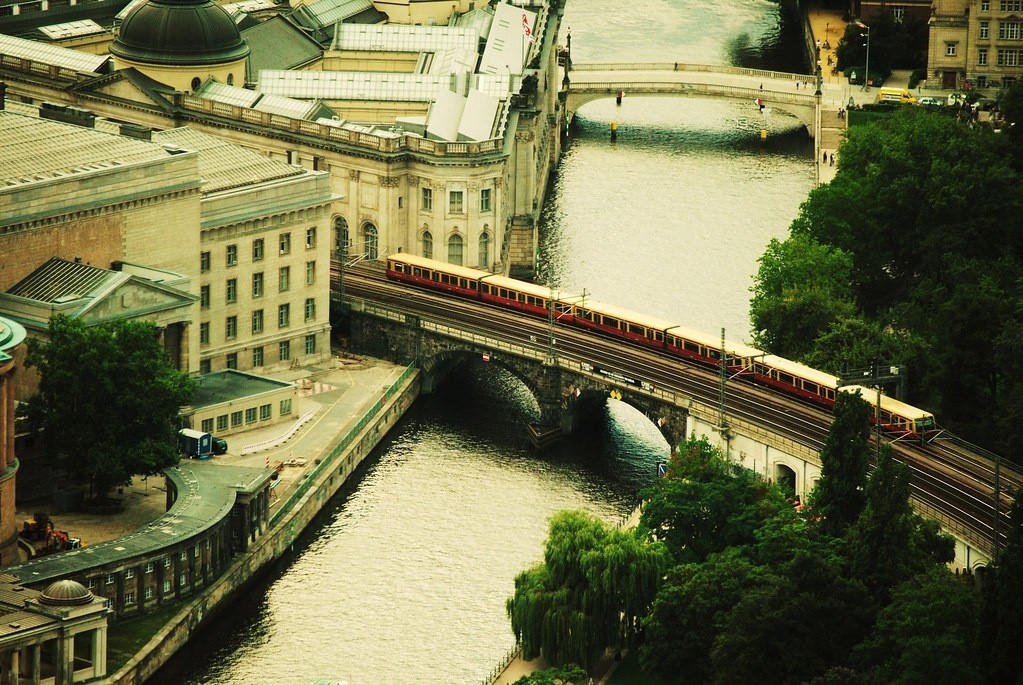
[212,436,227,456]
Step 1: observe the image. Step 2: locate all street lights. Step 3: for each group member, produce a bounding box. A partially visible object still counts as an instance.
[566,27,573,65]
[815,39,820,75]
[857,21,869,91]
[562,45,570,84]
[814,59,823,95]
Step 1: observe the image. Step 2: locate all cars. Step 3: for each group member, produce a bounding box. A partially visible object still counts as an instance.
[919,96,944,106]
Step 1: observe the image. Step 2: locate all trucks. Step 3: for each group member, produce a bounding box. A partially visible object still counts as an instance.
[880,86,917,105]
[177,427,212,460]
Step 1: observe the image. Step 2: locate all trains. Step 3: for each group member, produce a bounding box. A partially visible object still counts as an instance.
[386,250,937,439]
